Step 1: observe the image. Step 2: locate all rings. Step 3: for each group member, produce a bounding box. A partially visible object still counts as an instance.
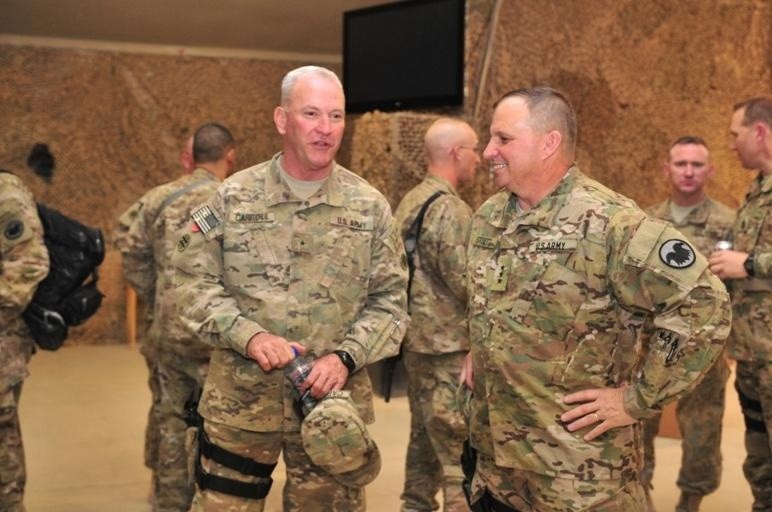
[594,414,598,423]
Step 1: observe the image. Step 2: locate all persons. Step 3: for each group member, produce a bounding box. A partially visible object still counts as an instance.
[708,98,772,512]
[122,122,237,512]
[390,117,483,512]
[461,86,733,511]
[0,168,51,511]
[173,64,413,511]
[632,136,738,511]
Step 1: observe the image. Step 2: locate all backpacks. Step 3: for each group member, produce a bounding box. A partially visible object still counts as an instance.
[1,168,105,352]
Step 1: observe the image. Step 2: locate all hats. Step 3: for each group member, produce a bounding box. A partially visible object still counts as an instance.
[300,389,382,491]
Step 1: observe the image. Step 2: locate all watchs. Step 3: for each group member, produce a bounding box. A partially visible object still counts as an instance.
[335,350,355,374]
[743,253,755,277]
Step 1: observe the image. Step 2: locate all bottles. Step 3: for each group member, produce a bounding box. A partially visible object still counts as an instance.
[284,347,318,416]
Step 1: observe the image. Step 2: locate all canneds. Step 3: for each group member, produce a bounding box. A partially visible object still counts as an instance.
[714,240,732,251]
